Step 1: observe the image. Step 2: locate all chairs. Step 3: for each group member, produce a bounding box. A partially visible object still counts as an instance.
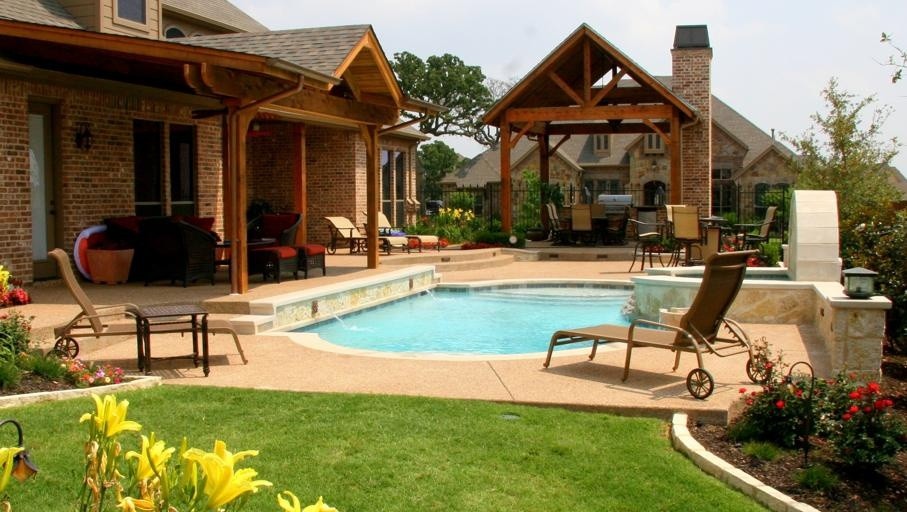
[627,203,778,272]
[546,202,631,248]
[247,210,302,280]
[544,247,772,399]
[323,211,440,254]
[104,215,220,287]
[48,248,248,364]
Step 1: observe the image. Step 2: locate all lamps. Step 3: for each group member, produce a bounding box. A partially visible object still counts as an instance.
[77,121,93,150]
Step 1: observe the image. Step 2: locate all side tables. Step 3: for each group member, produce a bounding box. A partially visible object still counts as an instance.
[130,304,212,377]
[86,249,135,285]
[215,240,231,284]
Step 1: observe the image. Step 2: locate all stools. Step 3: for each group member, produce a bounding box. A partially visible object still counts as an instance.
[248,244,325,284]
